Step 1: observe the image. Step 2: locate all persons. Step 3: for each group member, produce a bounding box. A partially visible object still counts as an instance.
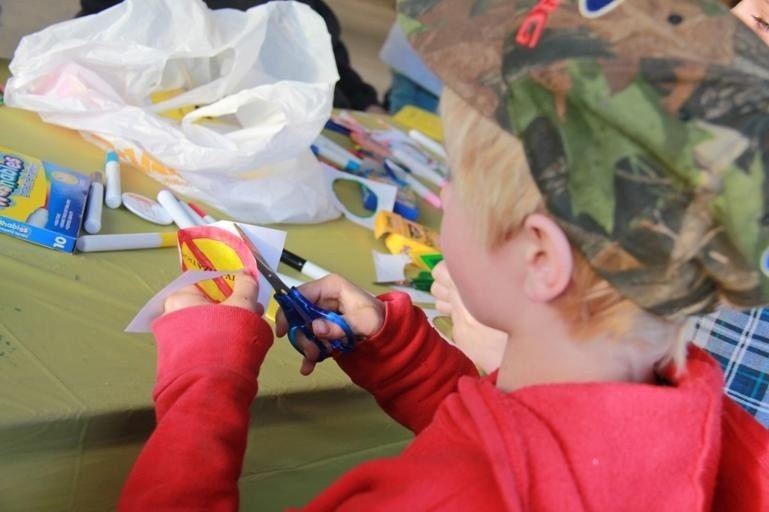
[74,0,390,114]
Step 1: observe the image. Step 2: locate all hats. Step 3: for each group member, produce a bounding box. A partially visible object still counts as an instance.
[395,0,768,326]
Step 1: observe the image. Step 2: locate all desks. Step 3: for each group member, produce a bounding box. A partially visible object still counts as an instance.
[1,58,618,428]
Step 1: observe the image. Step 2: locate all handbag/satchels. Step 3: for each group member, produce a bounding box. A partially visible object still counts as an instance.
[5,0,341,227]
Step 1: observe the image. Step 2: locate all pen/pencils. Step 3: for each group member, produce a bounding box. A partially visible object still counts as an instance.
[311,110,452,221]
[76,150,218,253]
[280,249,329,279]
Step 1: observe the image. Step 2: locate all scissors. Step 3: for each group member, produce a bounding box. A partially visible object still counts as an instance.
[232,223,356,363]
